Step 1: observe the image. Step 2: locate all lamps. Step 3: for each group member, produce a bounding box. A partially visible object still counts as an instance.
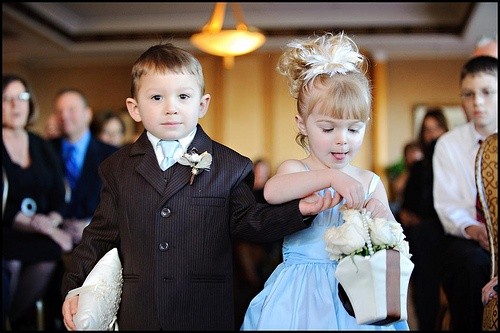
[189,2,267,68]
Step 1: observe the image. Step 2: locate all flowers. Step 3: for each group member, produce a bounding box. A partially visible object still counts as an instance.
[325,204,413,261]
[178,150,213,186]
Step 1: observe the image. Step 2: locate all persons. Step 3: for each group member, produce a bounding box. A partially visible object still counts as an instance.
[2,74,148,331]
[237,159,273,287]
[388,54,498,331]
[239,31,412,331]
[55,39,342,331]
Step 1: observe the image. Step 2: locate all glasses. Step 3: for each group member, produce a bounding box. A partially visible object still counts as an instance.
[0,90,31,101]
[460,87,497,97]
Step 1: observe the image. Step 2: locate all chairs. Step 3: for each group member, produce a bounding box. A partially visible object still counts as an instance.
[471,129,499,333]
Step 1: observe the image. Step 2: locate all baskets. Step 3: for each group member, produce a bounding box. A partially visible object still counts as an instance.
[335,206,415,327]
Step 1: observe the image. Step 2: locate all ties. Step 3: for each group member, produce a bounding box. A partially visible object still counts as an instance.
[62,143,80,193]
[158,139,179,171]
